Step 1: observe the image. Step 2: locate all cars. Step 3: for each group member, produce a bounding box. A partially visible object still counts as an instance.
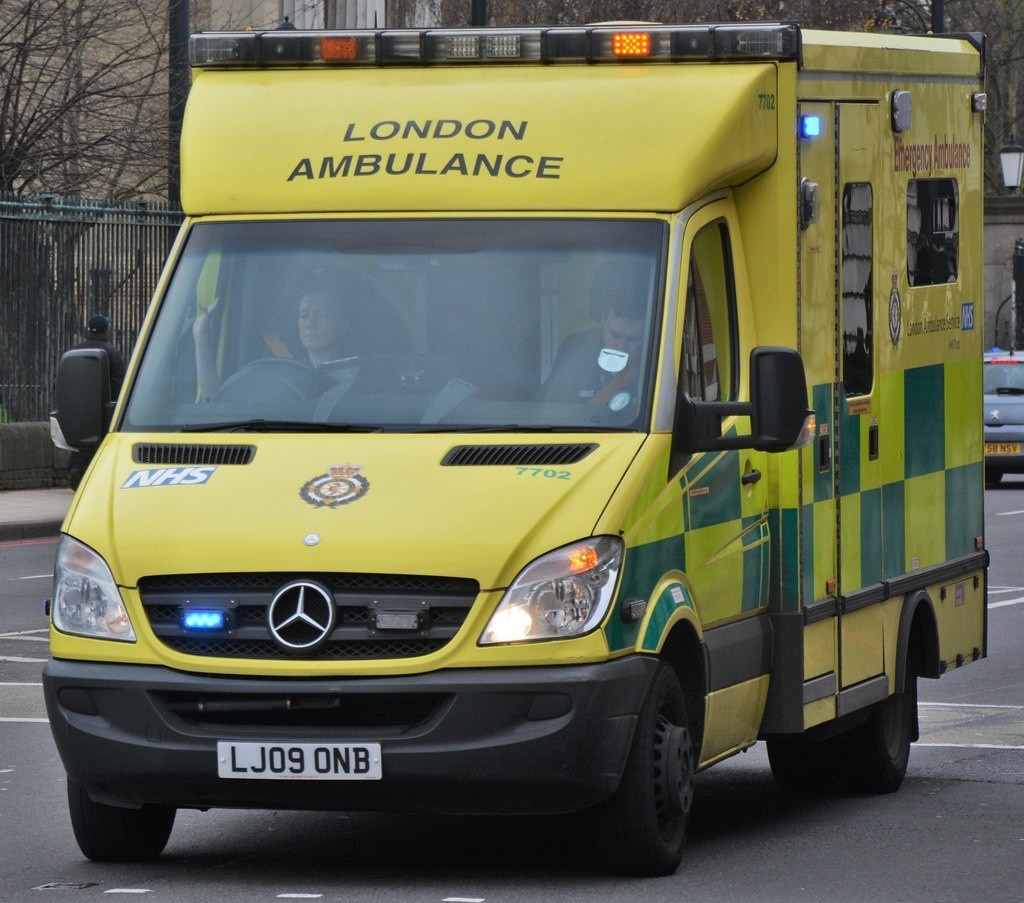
[984,342,1024,482]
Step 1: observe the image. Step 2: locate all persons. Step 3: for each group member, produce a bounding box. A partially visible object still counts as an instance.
[537,293,649,407]
[68,315,126,494]
[193,280,352,405]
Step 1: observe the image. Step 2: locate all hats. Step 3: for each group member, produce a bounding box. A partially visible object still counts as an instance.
[89,316,108,334]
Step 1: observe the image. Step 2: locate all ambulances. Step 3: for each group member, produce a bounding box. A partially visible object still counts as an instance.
[44,19,990,878]
